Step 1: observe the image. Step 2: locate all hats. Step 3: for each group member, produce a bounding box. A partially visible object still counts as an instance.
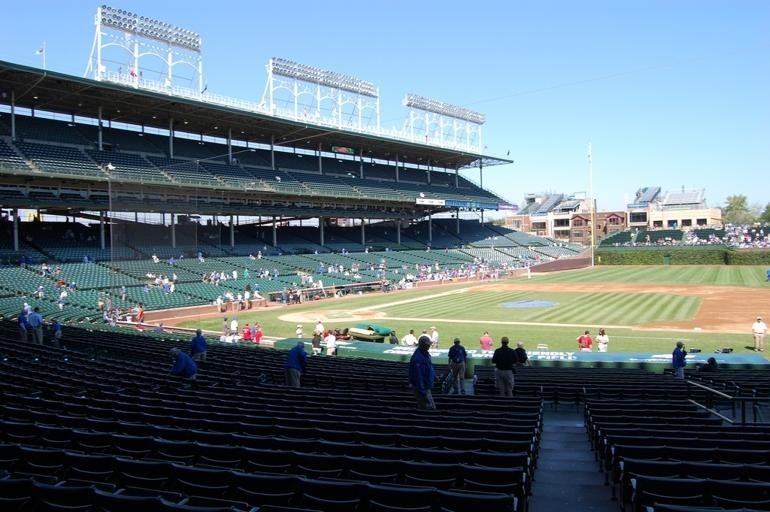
[454,338,461,343]
[419,336,432,346]
[501,338,508,343]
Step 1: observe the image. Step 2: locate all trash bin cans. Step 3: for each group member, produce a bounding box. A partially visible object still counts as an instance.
[663,256,670,265]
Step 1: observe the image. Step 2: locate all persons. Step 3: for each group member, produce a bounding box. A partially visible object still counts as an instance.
[766,266,770,283]
[671,341,689,380]
[577,328,609,353]
[751,316,768,352]
[339,222,563,288]
[612,218,770,251]
[17,223,338,392]
[386,325,527,410]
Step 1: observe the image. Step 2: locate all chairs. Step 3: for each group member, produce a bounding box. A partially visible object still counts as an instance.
[0,222,587,325]
[0,326,766,511]
[0,140,513,207]
